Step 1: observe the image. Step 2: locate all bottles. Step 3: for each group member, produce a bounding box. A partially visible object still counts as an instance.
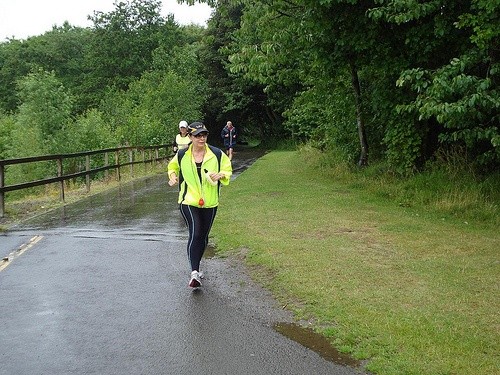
[204,169,218,186]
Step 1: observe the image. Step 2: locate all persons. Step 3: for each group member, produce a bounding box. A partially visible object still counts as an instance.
[166,121,232,288]
[172,121,193,153]
[221,121,236,161]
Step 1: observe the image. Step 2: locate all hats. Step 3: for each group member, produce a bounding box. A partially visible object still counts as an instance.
[179,121,188,128]
[186,122,209,135]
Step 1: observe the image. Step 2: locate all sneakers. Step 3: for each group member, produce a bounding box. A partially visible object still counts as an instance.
[198,271,204,278]
[189,270,202,287]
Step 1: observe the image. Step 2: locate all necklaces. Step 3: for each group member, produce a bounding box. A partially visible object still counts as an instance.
[192,144,206,164]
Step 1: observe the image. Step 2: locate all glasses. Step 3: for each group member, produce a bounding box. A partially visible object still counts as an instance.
[193,134,208,139]
